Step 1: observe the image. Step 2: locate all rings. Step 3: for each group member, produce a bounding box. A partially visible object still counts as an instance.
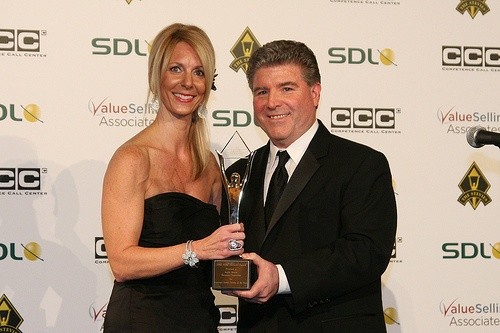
[228,239,243,251]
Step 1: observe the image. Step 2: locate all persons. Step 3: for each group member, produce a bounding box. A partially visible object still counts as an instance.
[220,40,398,333]
[101,24,246,333]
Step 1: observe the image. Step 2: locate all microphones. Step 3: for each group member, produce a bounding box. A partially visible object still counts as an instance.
[466,126,500,149]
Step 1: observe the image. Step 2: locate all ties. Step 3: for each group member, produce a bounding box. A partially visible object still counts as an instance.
[264,150,291,236]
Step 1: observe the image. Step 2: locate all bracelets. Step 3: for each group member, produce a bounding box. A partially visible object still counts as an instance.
[182,240,200,269]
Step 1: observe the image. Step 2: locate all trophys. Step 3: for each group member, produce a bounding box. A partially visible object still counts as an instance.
[212,149,258,291]
